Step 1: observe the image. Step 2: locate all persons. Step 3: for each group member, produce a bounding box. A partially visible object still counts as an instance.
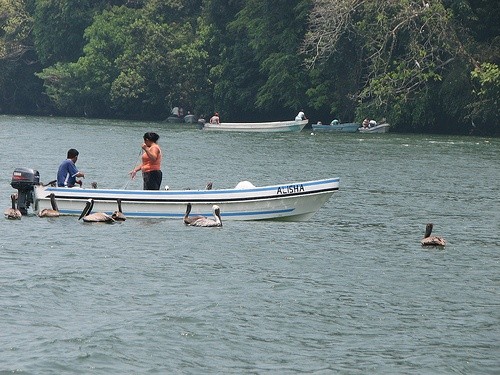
[57,149,85,187]
[210,112,220,125]
[295,109,307,121]
[317,118,386,129]
[171,104,184,119]
[128,132,162,190]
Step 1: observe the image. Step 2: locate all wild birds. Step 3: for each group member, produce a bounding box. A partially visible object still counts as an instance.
[112,198,126,221]
[184,203,222,227]
[78,198,115,221]
[205,182,212,190]
[421,224,446,246]
[39,193,60,218]
[4,194,22,219]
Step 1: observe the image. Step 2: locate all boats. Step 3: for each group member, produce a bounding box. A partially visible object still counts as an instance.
[204,118,309,134]
[312,122,361,132]
[9,168,340,221]
[358,123,390,133]
